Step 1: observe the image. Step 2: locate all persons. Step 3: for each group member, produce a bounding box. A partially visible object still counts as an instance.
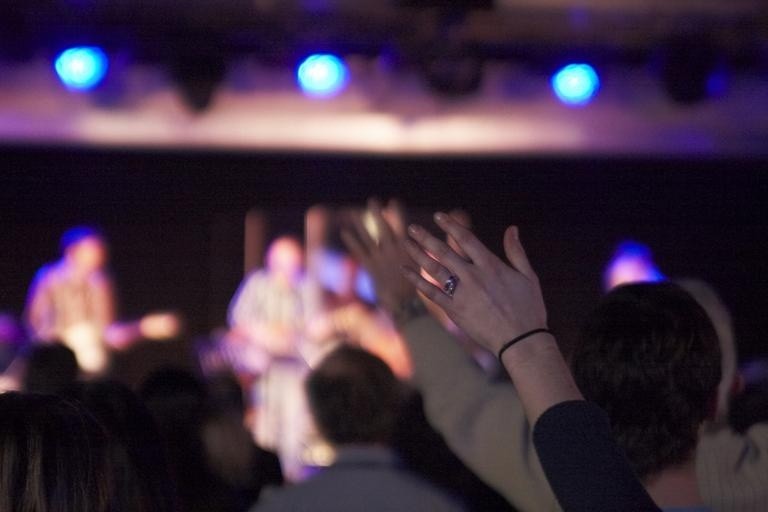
[1,187,766,509]
